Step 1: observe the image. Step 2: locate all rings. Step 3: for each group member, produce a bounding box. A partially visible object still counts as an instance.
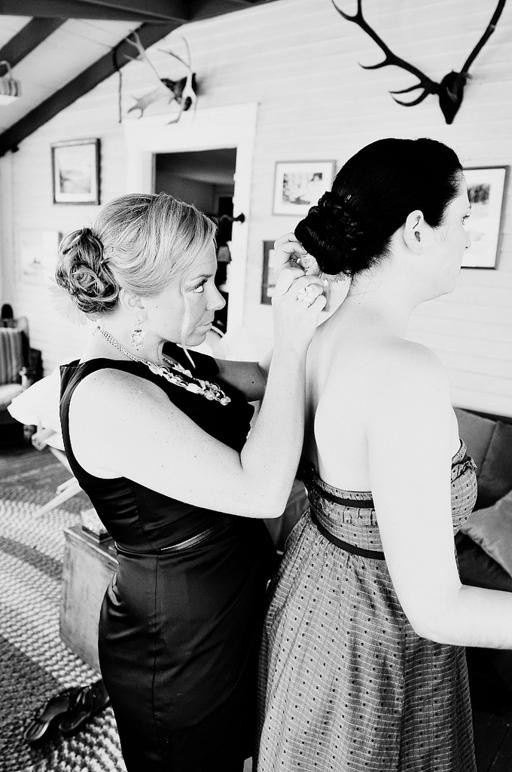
[297,286,316,304]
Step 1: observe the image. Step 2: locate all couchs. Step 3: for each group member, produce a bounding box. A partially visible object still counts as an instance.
[0,327,45,452]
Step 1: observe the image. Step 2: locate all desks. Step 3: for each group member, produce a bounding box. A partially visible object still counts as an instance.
[58,477,310,676]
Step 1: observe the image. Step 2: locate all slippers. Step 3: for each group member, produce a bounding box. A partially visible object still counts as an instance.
[23,679,112,750]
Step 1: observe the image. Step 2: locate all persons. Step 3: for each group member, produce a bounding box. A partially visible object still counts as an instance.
[252,136,512,771]
[53,191,329,772]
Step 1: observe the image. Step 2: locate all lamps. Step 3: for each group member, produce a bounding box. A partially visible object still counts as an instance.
[1,60,20,105]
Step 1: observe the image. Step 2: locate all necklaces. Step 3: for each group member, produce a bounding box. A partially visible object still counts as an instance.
[99,321,235,407]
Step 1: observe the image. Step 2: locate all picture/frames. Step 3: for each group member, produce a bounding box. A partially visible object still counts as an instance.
[460,164,510,272]
[50,136,102,206]
[271,159,337,217]
